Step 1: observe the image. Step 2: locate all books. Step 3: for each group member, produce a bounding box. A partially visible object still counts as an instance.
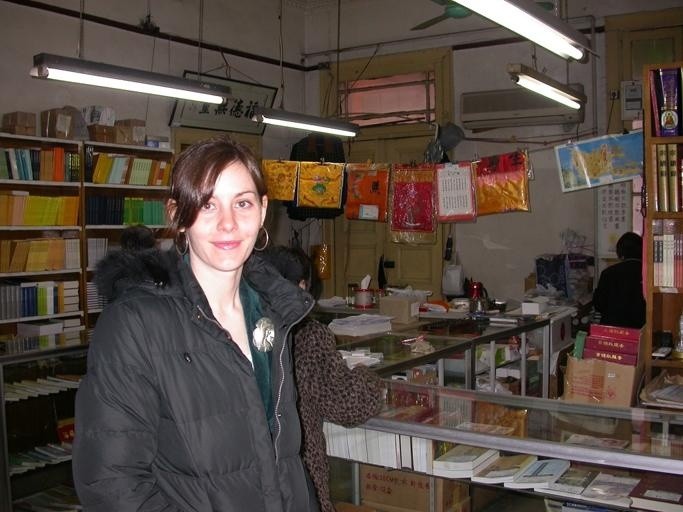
[158,238,173,251]
[0,230,81,320]
[653,383,683,407]
[379,406,460,422]
[84,146,174,226]
[488,317,520,324]
[87,238,108,309]
[393,362,439,385]
[581,348,638,368]
[9,417,83,511]
[495,356,539,379]
[585,335,639,355]
[323,422,683,512]
[648,69,682,295]
[1,318,94,401]
[488,323,520,328]
[1,146,80,226]
[590,323,641,341]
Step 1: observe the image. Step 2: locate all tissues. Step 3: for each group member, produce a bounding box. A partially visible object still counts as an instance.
[353,274,375,308]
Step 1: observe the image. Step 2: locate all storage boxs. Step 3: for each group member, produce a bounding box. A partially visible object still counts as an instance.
[1,106,169,147]
[356,462,469,507]
[562,353,639,407]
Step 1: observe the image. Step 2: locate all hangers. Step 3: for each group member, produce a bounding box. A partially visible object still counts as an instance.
[537,234,596,264]
[310,131,325,146]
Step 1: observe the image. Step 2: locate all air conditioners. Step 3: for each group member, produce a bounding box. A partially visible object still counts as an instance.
[459,80,585,132]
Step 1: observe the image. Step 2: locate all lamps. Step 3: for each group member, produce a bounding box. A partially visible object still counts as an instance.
[29,1,235,105]
[451,0,594,109]
[250,0,363,139]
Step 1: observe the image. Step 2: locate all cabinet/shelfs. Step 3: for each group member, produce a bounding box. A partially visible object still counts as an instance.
[0,132,176,500]
[640,59,682,457]
[331,302,577,398]
[325,379,680,511]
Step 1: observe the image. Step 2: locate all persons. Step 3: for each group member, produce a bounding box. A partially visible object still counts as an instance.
[253,240,388,510]
[68,130,322,512]
[589,230,647,330]
[118,223,156,253]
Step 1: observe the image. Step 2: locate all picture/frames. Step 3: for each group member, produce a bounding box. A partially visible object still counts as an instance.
[167,70,279,136]
[594,179,634,262]
